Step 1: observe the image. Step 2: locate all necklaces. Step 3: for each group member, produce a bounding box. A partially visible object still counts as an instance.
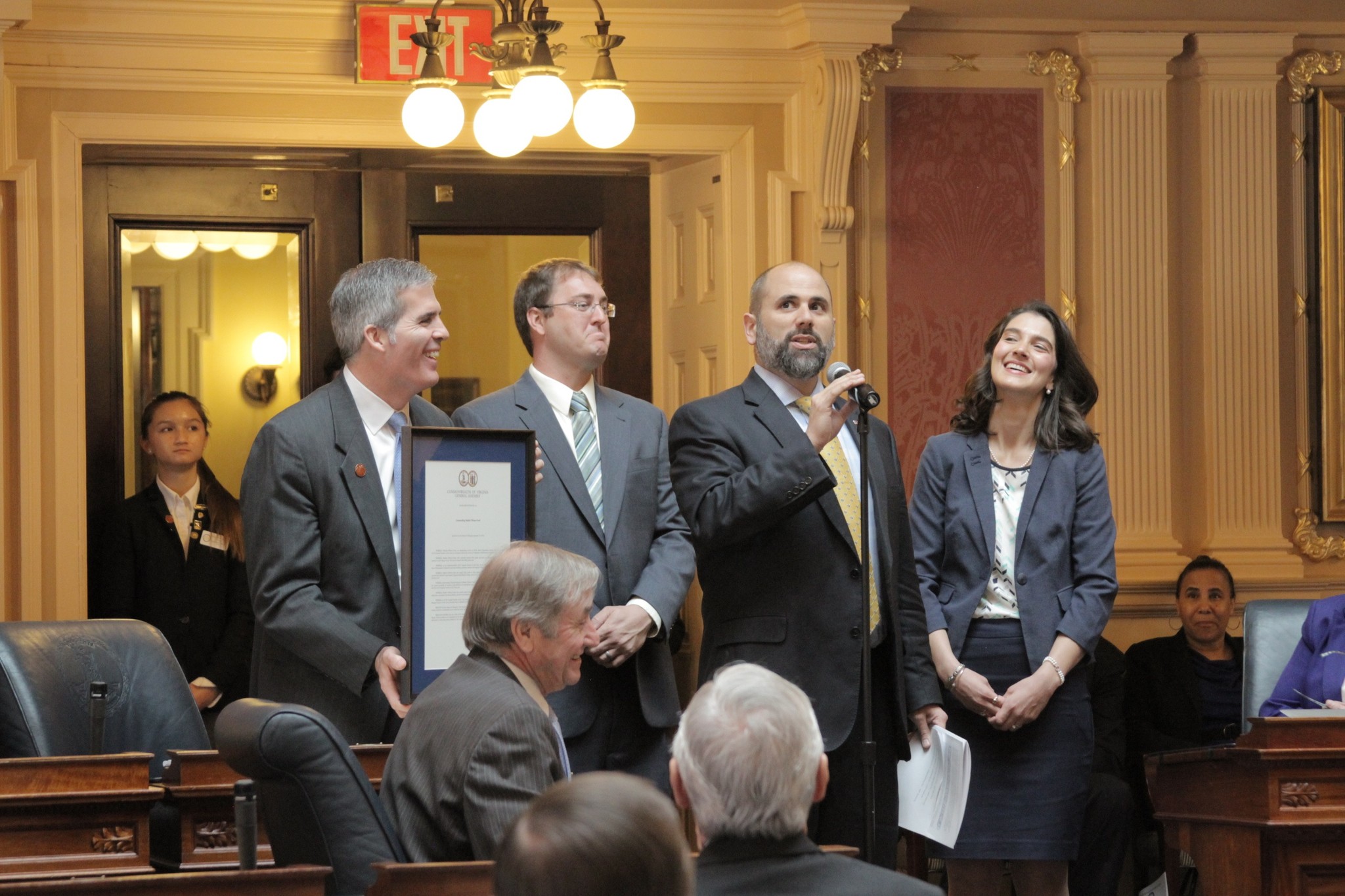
[987,443,1035,468]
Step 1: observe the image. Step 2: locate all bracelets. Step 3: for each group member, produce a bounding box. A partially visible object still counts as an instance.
[945,665,966,691]
[1042,655,1065,686]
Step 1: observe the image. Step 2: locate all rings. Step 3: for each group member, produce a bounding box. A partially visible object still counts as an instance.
[992,695,998,704]
[1012,724,1020,730]
[980,710,988,717]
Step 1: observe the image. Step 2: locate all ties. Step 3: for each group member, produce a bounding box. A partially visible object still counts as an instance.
[548,706,571,780]
[388,411,409,548]
[570,392,605,533]
[795,396,881,635]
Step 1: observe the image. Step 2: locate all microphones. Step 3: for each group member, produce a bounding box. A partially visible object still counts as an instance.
[827,362,880,409]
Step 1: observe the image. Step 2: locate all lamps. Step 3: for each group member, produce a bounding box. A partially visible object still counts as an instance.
[241,332,287,405]
[402,0,636,158]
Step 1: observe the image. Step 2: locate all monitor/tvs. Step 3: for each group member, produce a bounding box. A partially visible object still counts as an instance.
[1241,598,1320,732]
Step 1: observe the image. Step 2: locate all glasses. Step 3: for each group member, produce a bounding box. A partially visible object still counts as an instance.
[536,301,616,318]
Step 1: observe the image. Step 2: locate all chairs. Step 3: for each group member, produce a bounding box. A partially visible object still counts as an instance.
[212,695,410,896]
[1239,599,1317,732]
[0,617,212,758]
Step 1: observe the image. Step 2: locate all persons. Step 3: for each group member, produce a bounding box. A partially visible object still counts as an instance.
[95,391,260,753]
[665,658,949,896]
[1117,555,1244,766]
[905,294,1120,896]
[667,261,950,885]
[1259,592,1345,719]
[458,256,697,834]
[491,769,698,895]
[378,539,603,865]
[239,256,464,739]
